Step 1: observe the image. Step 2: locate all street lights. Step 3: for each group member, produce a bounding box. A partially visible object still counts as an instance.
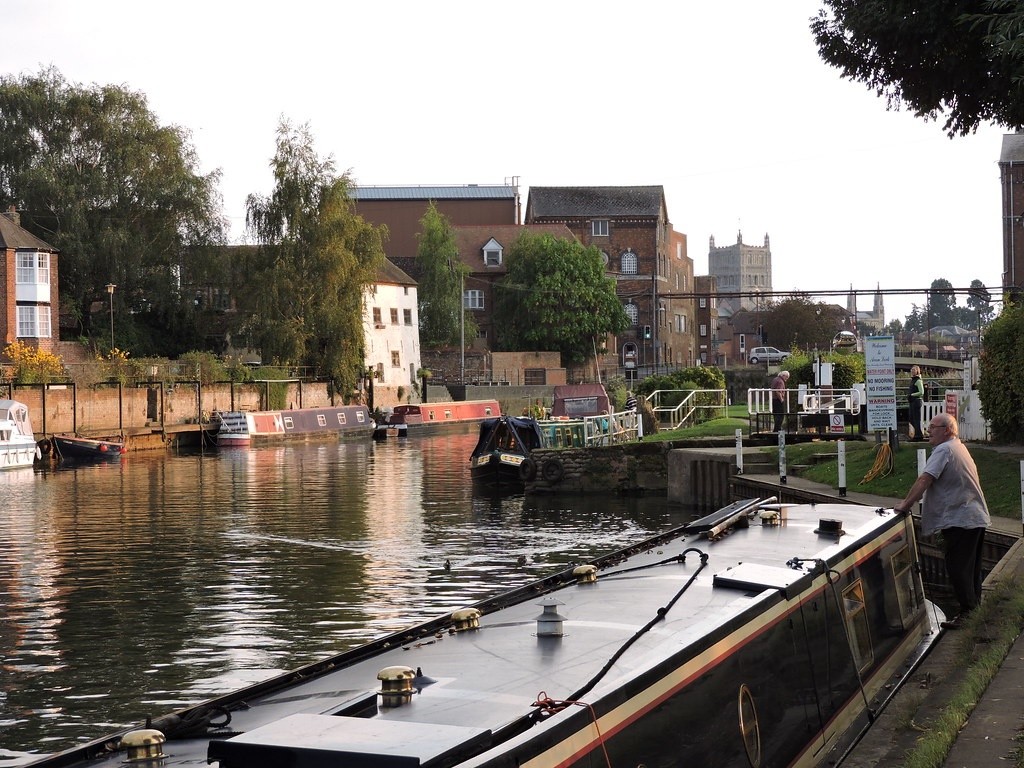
[105,282,117,362]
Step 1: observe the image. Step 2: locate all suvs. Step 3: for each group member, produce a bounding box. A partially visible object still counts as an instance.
[745,347,792,365]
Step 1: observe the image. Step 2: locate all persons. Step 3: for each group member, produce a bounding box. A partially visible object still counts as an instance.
[771,371,789,432]
[860,373,867,384]
[893,413,990,630]
[597,409,608,435]
[907,366,925,441]
[625,391,637,415]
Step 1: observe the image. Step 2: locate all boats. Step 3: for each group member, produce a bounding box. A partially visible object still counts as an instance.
[467,417,593,484]
[388,399,503,436]
[49,434,127,459]
[0,400,38,470]
[11,494,945,767]
[215,405,377,449]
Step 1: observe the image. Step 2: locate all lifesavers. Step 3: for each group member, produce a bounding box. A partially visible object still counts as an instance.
[519,459,537,481]
[542,459,564,484]
[550,416,569,420]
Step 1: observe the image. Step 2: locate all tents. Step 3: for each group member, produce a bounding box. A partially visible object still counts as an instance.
[472,416,543,464]
[554,382,608,416]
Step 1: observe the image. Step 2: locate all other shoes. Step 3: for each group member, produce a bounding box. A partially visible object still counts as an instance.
[907,437,918,442]
[918,437,923,441]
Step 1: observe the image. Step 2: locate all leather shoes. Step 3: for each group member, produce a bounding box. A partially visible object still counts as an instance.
[941,618,965,629]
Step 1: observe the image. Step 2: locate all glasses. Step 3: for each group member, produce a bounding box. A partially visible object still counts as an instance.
[928,424,946,429]
